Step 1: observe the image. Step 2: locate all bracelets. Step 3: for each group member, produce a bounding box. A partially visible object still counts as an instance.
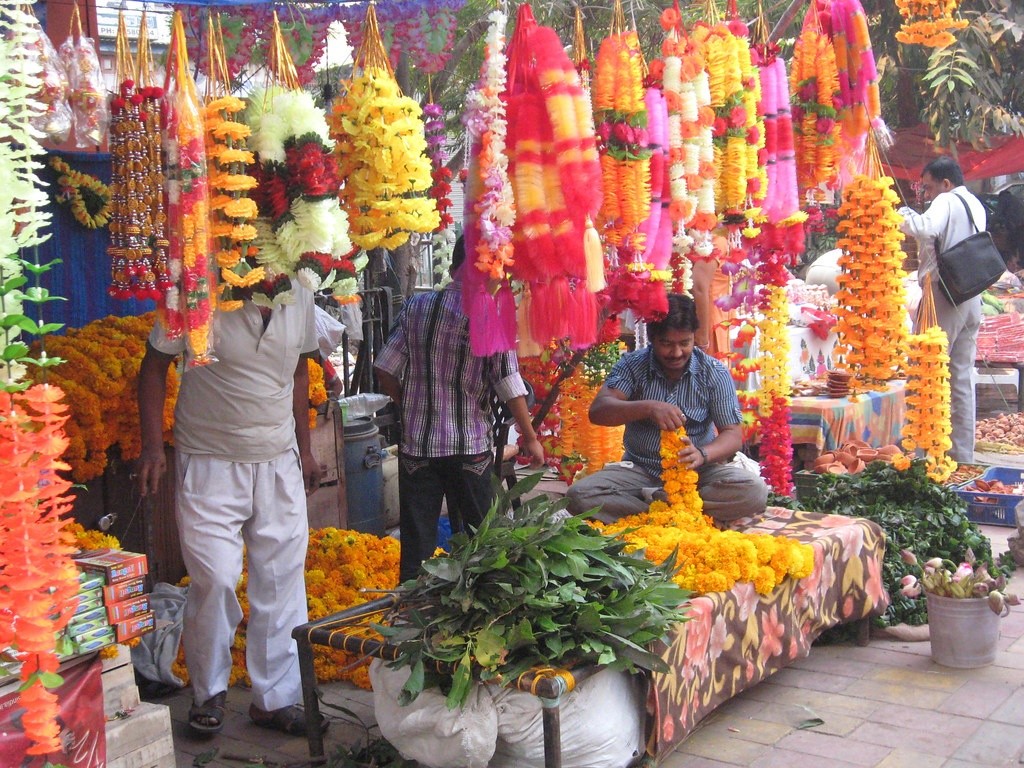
[694,340,710,351]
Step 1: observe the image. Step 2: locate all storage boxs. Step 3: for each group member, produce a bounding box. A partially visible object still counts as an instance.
[107,593,150,625]
[102,575,145,605]
[73,550,148,584]
[946,462,1024,528]
[794,469,819,498]
[114,610,156,643]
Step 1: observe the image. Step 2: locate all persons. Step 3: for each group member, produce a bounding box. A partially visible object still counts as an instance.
[564,295,767,525]
[806,249,850,292]
[895,157,987,462]
[375,235,545,585]
[987,190,1024,269]
[137,278,330,735]
[692,213,745,373]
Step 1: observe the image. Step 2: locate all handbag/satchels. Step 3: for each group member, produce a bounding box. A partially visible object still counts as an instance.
[933,194,1006,308]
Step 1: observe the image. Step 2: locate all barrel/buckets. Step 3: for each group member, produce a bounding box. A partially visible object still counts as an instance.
[341,424,387,539]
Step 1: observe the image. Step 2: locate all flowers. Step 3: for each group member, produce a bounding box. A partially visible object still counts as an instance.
[901,546,1022,615]
[0,0,968,756]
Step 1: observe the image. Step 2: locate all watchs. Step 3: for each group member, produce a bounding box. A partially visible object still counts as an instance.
[697,446,708,465]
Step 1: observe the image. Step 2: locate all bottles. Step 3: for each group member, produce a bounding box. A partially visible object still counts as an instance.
[336,393,396,424]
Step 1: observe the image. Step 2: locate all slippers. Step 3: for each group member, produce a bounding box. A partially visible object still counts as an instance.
[252,706,331,735]
[189,690,226,733]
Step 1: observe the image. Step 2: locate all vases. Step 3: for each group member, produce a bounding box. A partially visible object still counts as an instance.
[926,591,1001,667]
